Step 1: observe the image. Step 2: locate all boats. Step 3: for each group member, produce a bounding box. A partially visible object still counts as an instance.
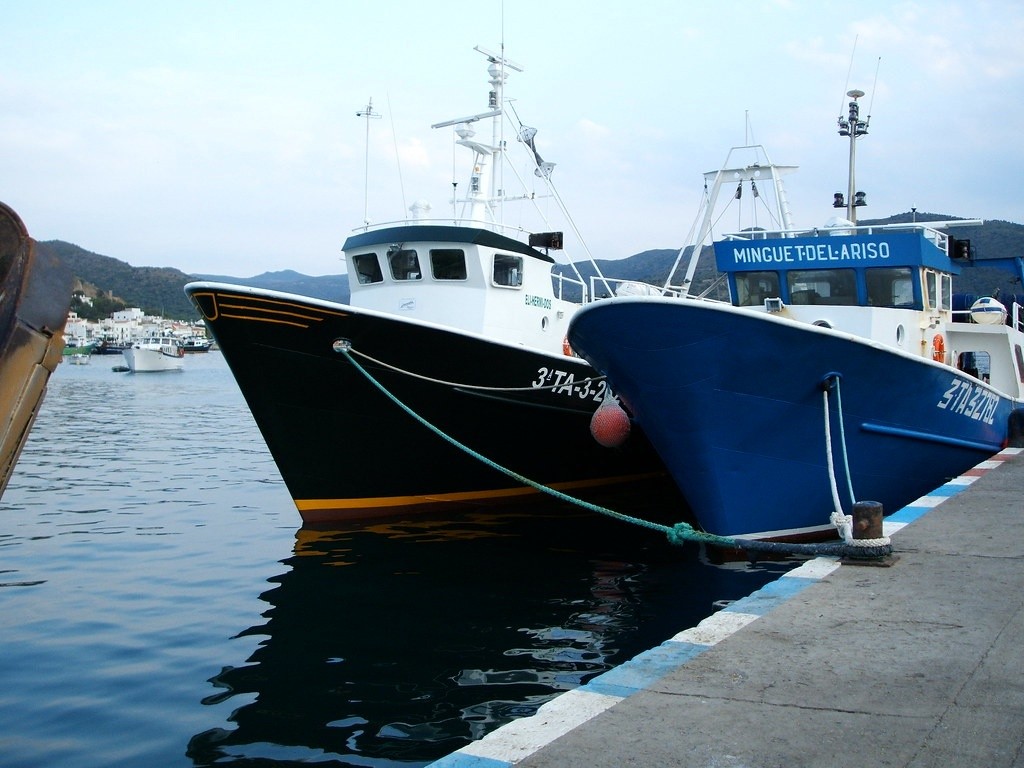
[180,42,800,531]
[1,196,77,518]
[122,323,183,374]
[161,344,184,358]
[177,340,213,351]
[68,354,89,364]
[567,34,1024,568]
[130,337,180,352]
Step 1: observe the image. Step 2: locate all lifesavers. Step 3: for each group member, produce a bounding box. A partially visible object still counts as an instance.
[931,333,946,363]
